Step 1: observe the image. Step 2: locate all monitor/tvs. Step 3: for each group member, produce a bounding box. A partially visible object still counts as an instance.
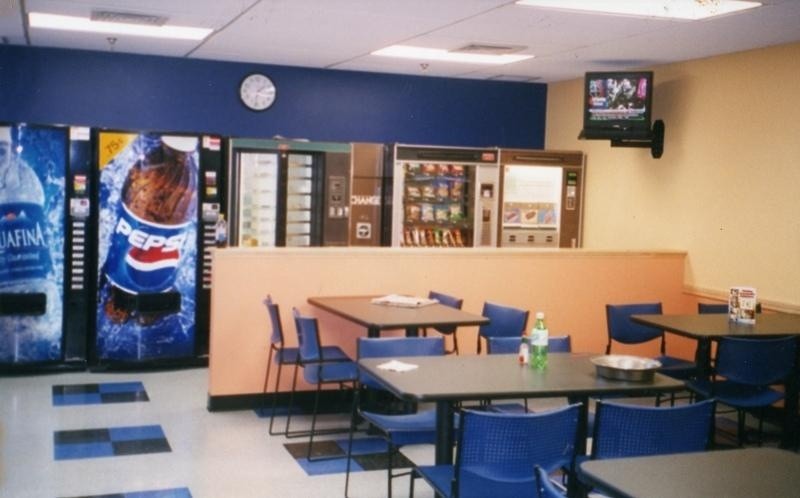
[584,70,652,131]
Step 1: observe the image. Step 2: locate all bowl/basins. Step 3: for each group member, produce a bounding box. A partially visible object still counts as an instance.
[591,356,663,383]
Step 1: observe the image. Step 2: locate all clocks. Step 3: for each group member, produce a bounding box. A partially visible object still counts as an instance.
[239,73,276,110]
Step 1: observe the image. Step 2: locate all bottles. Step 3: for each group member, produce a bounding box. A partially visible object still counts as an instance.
[95,135,200,325]
[531,312,549,369]
[518,334,529,366]
[0,125,63,363]
